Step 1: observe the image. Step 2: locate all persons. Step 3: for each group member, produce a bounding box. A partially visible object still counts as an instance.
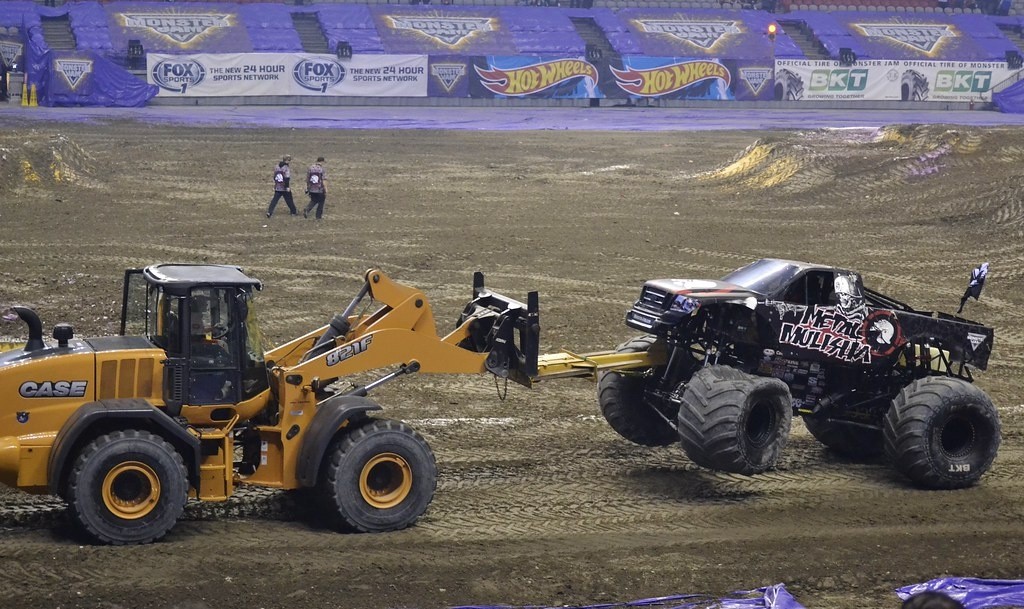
[413,0,450,5]
[295,0,303,5]
[570,0,593,8]
[938,0,977,9]
[304,157,327,219]
[174,302,228,379]
[531,0,559,6]
[266,155,299,218]
[731,0,776,13]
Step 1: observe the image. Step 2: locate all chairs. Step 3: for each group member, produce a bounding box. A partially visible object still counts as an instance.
[167,311,216,357]
[432,0,981,15]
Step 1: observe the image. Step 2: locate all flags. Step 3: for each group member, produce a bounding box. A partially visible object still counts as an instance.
[958,263,989,314]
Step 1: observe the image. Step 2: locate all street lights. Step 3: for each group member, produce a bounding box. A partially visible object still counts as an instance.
[769,25,775,58]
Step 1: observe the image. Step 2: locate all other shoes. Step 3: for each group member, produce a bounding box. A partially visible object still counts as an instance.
[291,213,298,216]
[266,212,271,219]
[304,208,308,218]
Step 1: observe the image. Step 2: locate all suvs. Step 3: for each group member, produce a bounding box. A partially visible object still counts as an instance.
[599,258,1003,491]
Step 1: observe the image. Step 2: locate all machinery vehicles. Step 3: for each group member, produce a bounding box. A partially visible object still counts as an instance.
[0,256,699,550]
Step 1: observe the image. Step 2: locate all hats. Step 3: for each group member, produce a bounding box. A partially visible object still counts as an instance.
[317,157,324,162]
[283,155,292,161]
[193,295,212,303]
